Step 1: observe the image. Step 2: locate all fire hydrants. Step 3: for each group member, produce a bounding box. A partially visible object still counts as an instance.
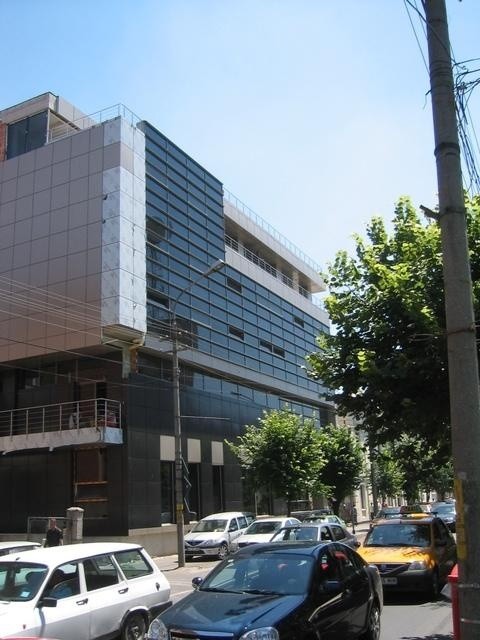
[448,564,460,640]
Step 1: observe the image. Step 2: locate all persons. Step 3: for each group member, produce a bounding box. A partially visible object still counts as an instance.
[43,518,64,547]
[98,409,118,427]
[42,569,73,600]
[69,408,87,430]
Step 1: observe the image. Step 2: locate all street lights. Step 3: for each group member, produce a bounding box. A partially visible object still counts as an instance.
[172,259,226,568]
